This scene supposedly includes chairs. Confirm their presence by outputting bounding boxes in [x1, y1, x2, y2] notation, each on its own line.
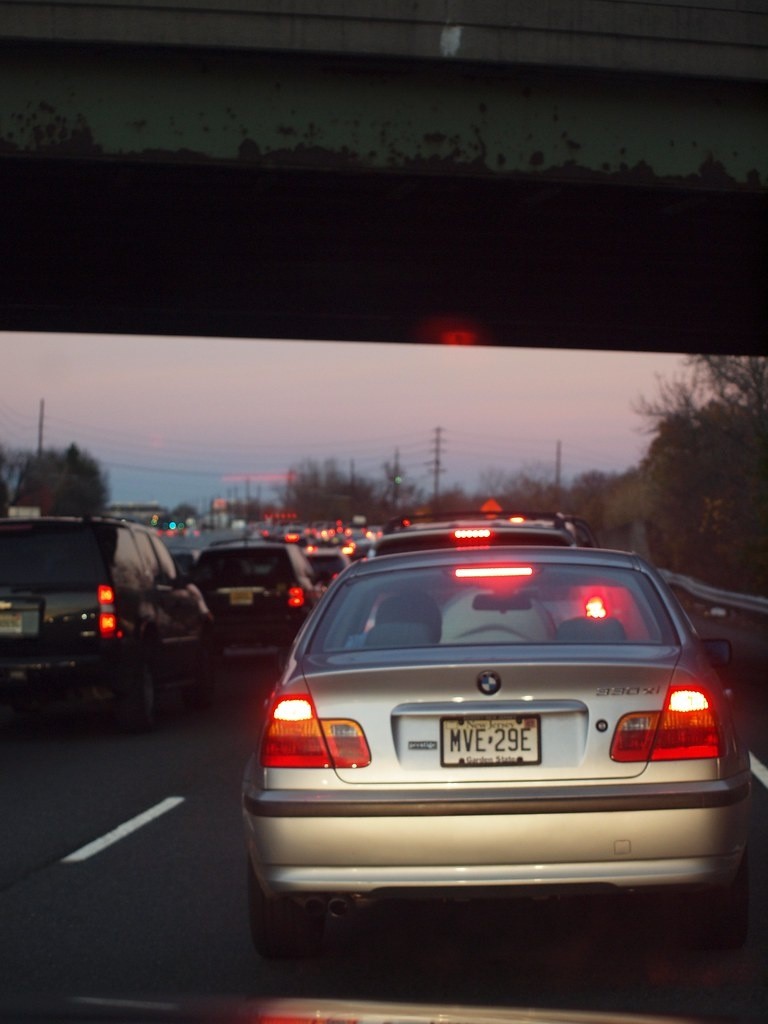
[555, 615, 627, 642]
[376, 592, 443, 646]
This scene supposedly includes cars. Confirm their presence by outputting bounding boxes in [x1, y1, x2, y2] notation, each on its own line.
[233, 543, 755, 947]
[112, 504, 589, 580]
[193, 542, 325, 666]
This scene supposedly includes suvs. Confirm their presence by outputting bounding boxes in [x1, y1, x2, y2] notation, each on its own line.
[2, 516, 218, 736]
